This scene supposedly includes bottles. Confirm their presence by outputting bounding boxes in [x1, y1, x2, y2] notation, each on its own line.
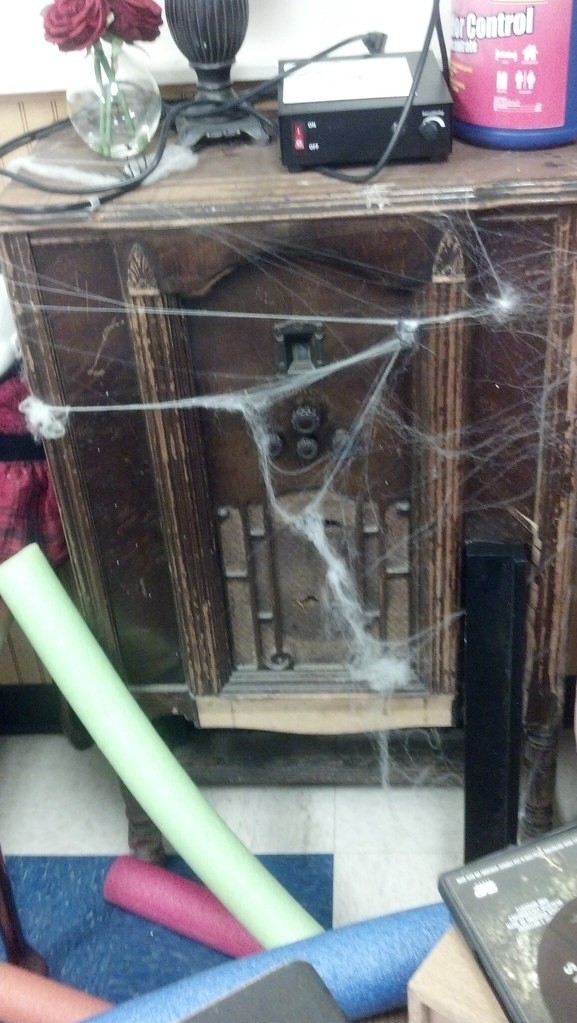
[442, 0, 577, 149]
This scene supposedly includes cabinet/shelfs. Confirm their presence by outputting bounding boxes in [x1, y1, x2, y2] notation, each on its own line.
[0, 139, 576, 868]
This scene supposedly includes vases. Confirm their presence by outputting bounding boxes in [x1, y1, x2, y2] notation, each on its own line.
[61, 57, 160, 157]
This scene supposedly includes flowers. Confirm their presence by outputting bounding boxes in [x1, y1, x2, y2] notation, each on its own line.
[43, 0, 162, 55]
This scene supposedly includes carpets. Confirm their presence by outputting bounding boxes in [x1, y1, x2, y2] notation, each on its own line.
[0, 855, 333, 1023]
